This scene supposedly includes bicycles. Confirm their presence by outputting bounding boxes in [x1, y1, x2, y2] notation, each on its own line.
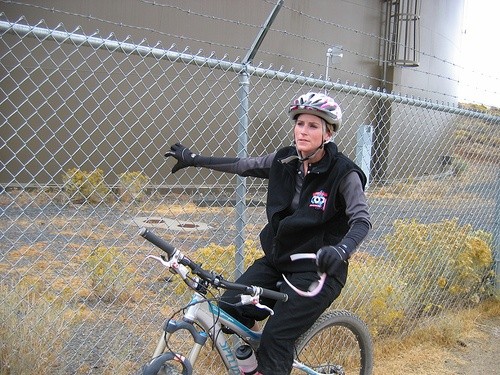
[134, 226, 374, 375]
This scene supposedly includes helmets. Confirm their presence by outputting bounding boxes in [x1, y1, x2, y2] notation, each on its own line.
[289, 92, 342, 132]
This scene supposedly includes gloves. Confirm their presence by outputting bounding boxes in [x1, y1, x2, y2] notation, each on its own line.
[316, 245, 347, 275]
[164, 143, 200, 174]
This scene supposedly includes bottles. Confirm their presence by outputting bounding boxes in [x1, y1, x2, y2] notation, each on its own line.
[235, 345, 258, 375]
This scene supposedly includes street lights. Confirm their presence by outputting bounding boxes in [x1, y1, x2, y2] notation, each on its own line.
[323, 44, 344, 95]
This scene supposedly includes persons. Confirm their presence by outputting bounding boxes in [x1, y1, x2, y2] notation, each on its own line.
[164, 92, 373, 375]
